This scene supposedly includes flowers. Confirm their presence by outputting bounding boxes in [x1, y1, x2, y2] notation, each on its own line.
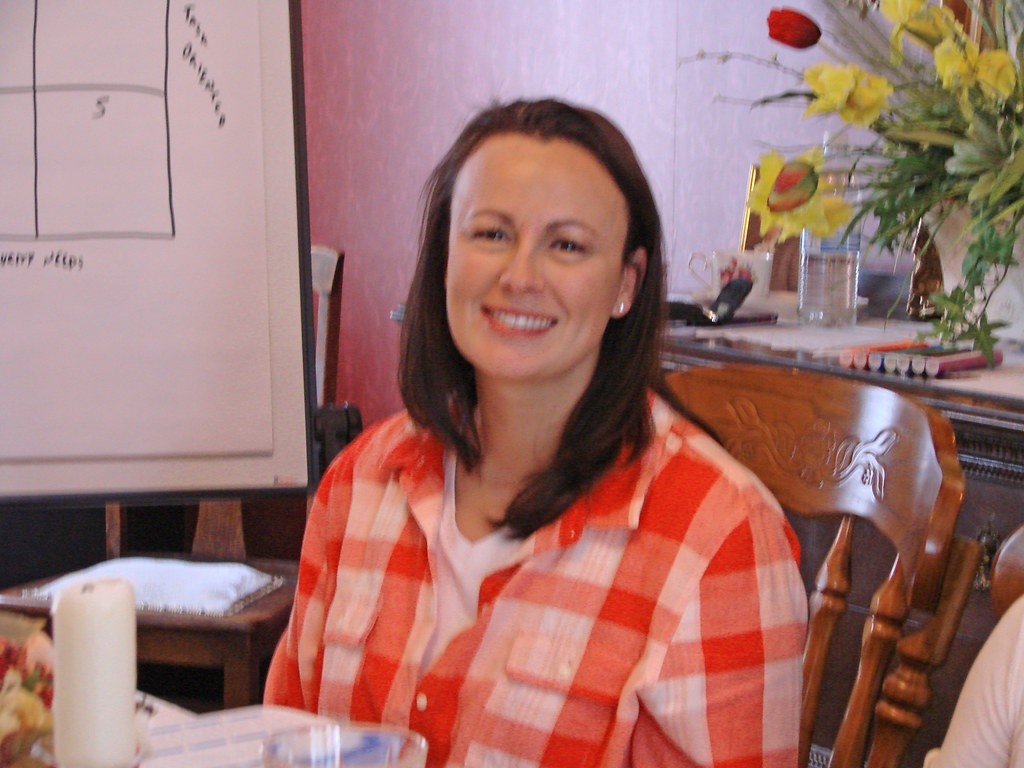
[749, 0, 1024, 373]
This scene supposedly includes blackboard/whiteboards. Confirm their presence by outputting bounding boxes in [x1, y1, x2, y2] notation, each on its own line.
[1, 0, 321, 504]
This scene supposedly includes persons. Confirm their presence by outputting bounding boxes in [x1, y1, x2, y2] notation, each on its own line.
[264, 97, 807, 768]
[930, 597, 1024, 768]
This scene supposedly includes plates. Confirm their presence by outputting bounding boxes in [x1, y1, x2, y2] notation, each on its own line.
[742, 290, 869, 319]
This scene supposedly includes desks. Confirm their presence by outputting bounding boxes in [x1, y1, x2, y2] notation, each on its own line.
[0, 560, 300, 711]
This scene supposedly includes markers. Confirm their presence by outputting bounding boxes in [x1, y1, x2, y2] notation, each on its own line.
[837, 339, 1002, 377]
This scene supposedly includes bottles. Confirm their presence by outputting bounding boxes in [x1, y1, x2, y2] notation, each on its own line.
[797, 130, 863, 327]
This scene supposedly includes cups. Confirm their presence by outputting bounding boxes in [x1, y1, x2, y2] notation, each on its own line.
[687, 249, 773, 298]
[260, 721, 429, 768]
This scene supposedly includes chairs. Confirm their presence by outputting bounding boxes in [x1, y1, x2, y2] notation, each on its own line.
[663, 366, 985, 768]
